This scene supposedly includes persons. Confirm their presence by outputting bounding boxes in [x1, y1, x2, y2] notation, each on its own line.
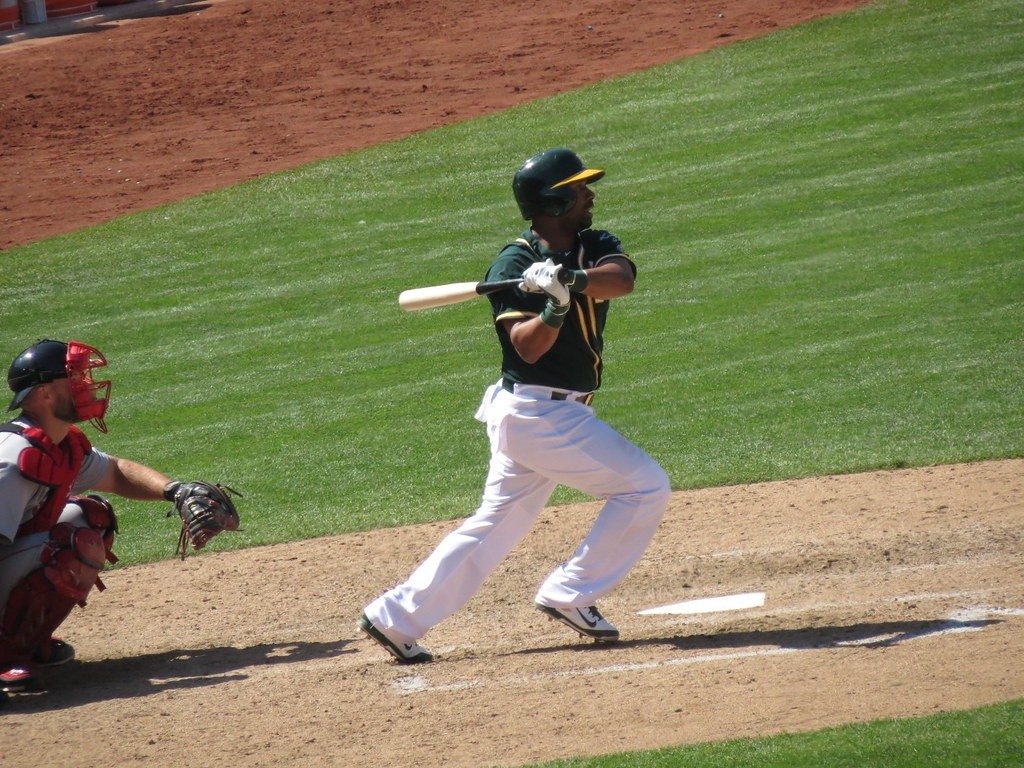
[354, 148, 671, 664]
[0, 338, 239, 700]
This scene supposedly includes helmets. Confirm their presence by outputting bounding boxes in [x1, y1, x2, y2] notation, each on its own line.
[7, 339, 83, 414]
[513, 147, 608, 220]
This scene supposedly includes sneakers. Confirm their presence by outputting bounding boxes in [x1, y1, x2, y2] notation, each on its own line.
[356, 610, 434, 663]
[535, 600, 620, 644]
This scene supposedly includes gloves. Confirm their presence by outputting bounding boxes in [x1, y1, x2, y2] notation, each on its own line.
[518, 260, 576, 296]
[534, 264, 571, 316]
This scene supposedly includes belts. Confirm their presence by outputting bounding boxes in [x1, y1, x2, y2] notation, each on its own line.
[502, 376, 594, 407]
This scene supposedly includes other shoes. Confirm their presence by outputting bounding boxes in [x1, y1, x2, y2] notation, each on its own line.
[21, 638, 75, 667]
[0, 663, 32, 692]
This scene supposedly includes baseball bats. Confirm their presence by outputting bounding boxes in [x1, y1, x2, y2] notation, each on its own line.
[397, 266, 573, 313]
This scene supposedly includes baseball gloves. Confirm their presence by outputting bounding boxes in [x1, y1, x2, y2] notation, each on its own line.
[166, 477, 241, 552]
